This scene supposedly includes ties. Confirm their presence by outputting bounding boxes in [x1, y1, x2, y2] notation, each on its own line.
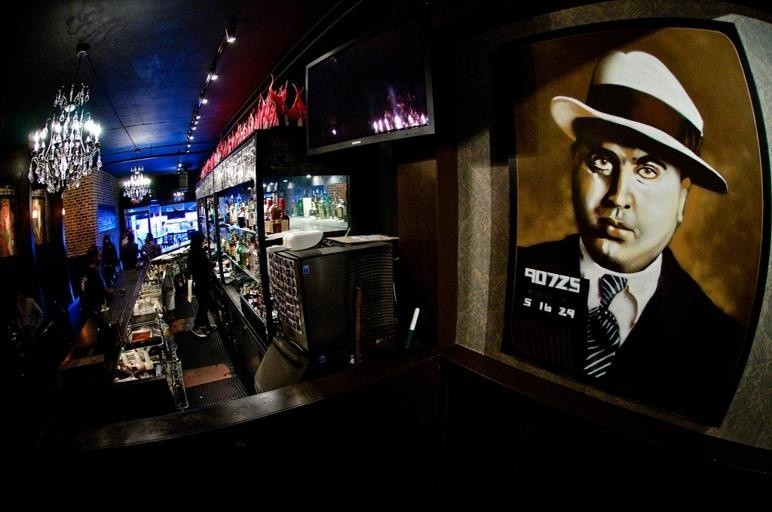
[584, 275, 628, 379]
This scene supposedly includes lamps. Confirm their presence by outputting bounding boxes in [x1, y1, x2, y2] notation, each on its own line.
[27, 42, 105, 196]
[176, 27, 237, 173]
[119, 148, 155, 206]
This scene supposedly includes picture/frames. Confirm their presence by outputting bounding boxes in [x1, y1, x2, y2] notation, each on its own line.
[497, 17, 772, 432]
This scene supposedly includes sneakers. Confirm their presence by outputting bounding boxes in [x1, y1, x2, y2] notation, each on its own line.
[192, 324, 216, 340]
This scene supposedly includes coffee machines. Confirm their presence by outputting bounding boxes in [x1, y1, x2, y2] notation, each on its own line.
[253, 221, 426, 398]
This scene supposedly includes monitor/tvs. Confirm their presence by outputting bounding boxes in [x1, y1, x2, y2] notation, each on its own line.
[303, 28, 439, 158]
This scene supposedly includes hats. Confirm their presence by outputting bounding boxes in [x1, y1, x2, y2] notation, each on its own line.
[550, 52, 730, 194]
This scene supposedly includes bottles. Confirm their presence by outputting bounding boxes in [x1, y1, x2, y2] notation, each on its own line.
[198, 176, 350, 325]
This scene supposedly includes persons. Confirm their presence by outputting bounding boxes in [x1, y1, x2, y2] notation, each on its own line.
[513, 47, 742, 426]
[81, 247, 112, 313]
[102, 234, 120, 284]
[16, 288, 46, 347]
[121, 227, 158, 267]
[183, 231, 220, 338]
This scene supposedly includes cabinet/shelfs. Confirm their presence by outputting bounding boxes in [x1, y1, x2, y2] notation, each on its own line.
[194, 124, 400, 397]
